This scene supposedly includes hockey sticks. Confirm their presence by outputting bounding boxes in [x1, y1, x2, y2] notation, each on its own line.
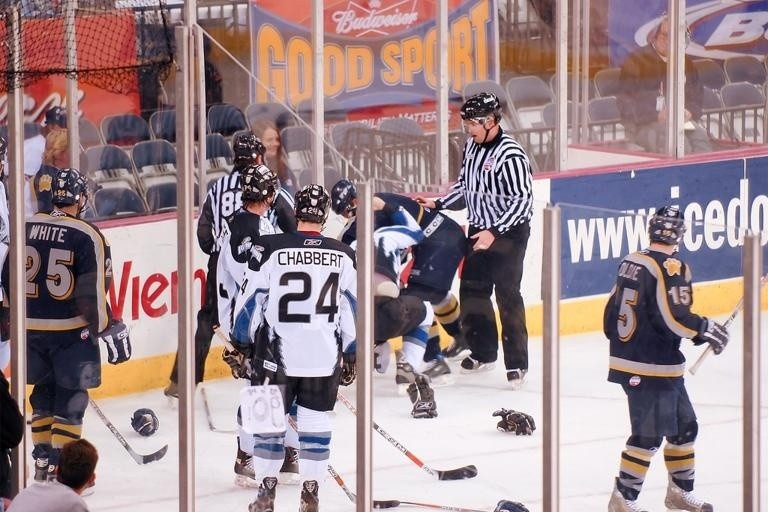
[88, 395, 168, 465]
[212, 324, 400, 508]
[201, 383, 240, 434]
[338, 393, 478, 482]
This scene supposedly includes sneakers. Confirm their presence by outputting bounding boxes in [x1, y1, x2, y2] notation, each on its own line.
[462, 354, 485, 370]
[233, 436, 258, 487]
[395, 338, 465, 418]
[279, 446, 301, 484]
[507, 369, 526, 380]
[248, 477, 278, 511]
[299, 480, 319, 511]
[165, 380, 179, 406]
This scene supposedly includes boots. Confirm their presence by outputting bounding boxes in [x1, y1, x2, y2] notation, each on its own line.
[608, 477, 647, 511]
[34, 457, 49, 480]
[665, 472, 712, 512]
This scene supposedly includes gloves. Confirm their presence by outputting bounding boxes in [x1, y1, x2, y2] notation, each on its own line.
[99, 319, 131, 364]
[691, 316, 728, 354]
[220, 344, 254, 380]
[493, 408, 536, 435]
[338, 361, 357, 386]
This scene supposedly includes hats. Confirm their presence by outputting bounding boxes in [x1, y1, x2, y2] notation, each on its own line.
[44, 107, 68, 129]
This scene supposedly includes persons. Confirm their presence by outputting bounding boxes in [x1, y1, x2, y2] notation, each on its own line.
[5, 439, 100, 512]
[615, 16, 712, 157]
[602, 205, 731, 512]
[223, 183, 360, 512]
[410, 91, 534, 381]
[161, 120, 467, 482]
[0, 370, 24, 512]
[1, 106, 131, 482]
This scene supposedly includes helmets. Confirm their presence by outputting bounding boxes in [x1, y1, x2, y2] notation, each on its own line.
[233, 93, 501, 224]
[648, 204, 687, 245]
[50, 168, 89, 204]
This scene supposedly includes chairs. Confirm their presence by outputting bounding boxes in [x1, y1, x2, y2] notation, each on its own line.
[0, 102, 246, 219]
[244, 95, 425, 200]
